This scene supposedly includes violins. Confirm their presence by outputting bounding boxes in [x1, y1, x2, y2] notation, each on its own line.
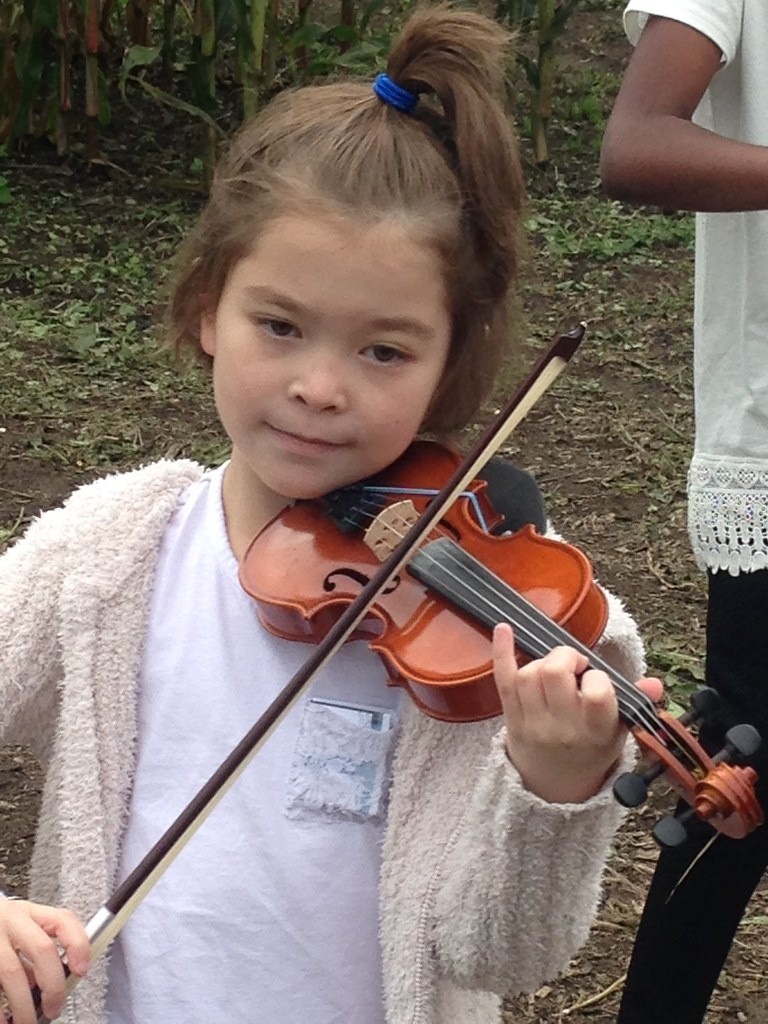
[235, 438, 767, 850]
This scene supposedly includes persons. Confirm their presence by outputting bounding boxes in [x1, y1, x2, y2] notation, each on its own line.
[0, 0, 666, 1023]
[588, 0, 768, 1024]
